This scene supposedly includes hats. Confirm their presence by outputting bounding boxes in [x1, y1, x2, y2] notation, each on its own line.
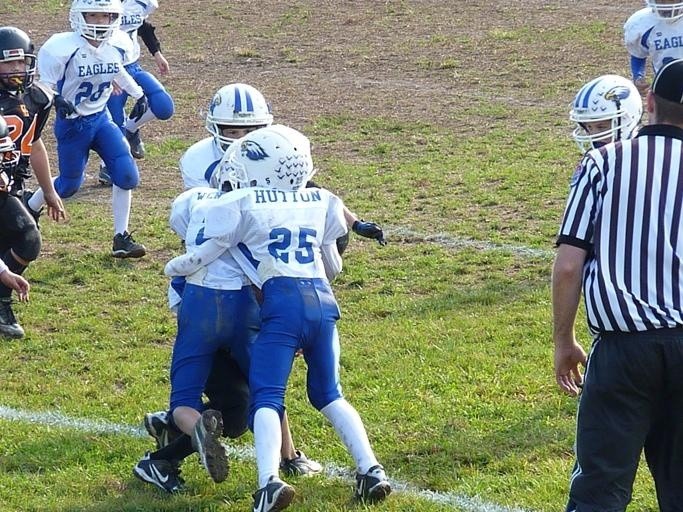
[652, 57, 683, 104]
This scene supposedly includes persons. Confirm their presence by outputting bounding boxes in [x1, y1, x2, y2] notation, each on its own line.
[0, 27, 66, 340]
[22, 0, 149, 257]
[98, 0, 174, 185]
[550, 61, 683, 512]
[0, 117, 30, 302]
[164, 124, 392, 512]
[169, 163, 322, 484]
[132, 82, 389, 493]
[623, 1, 682, 93]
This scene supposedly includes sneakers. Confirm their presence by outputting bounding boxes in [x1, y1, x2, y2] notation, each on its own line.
[112, 229, 147, 259]
[353, 462, 394, 507]
[250, 480, 297, 512]
[194, 407, 230, 485]
[124, 129, 146, 160]
[133, 456, 189, 499]
[281, 453, 327, 480]
[98, 161, 115, 187]
[144, 410, 174, 452]
[1, 302, 26, 340]
[19, 189, 40, 234]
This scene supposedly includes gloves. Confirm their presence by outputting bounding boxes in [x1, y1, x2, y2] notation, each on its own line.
[356, 221, 384, 246]
[129, 95, 149, 123]
[53, 94, 78, 120]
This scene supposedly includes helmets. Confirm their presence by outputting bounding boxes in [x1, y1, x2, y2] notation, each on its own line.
[0, 27, 37, 92]
[204, 84, 275, 152]
[70, 0, 125, 43]
[0, 116, 21, 189]
[646, 0, 683, 22]
[235, 124, 314, 189]
